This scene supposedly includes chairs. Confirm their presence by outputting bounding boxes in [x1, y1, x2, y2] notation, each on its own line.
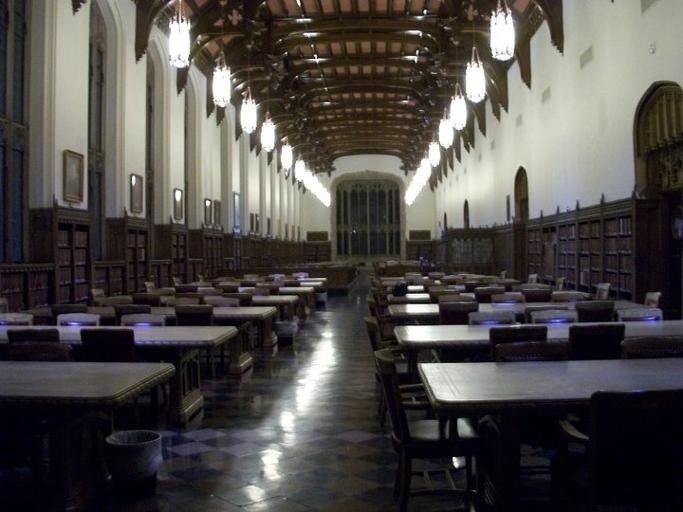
[165, 0, 308, 189]
[429, 0, 518, 176]
[1, 269, 328, 512]
[364, 255, 683, 511]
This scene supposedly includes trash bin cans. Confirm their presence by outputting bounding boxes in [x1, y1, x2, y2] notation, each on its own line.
[105, 429, 162, 500]
[313, 292, 329, 308]
[275, 321, 298, 346]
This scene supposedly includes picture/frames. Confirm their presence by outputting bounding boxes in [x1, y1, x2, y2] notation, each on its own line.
[62, 152, 302, 243]
